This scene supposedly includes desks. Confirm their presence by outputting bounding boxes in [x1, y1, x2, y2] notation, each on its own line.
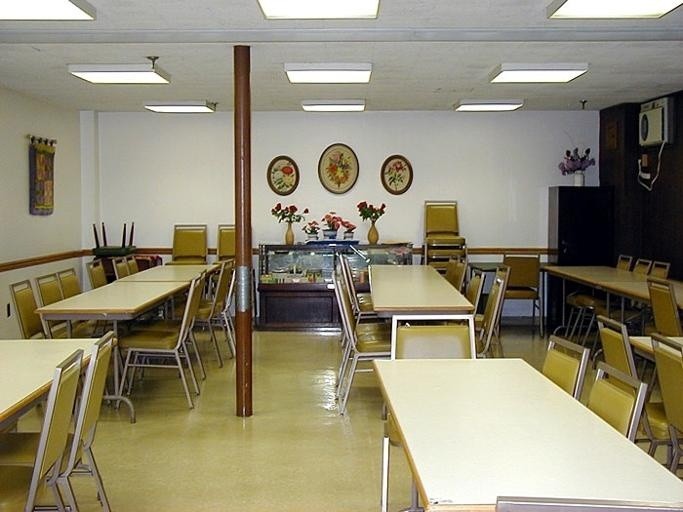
[1, 339, 118, 430]
[373, 358, 682, 511]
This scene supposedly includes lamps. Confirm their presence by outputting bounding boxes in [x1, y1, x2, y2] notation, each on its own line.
[66, 62, 171, 86]
[0, 1, 98, 23]
[299, 99, 366, 113]
[141, 99, 215, 114]
[281, 61, 373, 86]
[545, 0, 683, 21]
[254, 0, 381, 22]
[452, 98, 524, 112]
[487, 62, 589, 86]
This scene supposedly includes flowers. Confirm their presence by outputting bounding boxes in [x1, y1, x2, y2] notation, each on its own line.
[320, 210, 357, 233]
[557, 145, 595, 176]
[271, 165, 294, 192]
[384, 161, 408, 191]
[269, 202, 308, 224]
[324, 152, 352, 188]
[300, 221, 319, 236]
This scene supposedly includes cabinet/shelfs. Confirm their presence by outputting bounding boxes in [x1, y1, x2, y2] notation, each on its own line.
[255, 242, 414, 331]
[545, 183, 614, 337]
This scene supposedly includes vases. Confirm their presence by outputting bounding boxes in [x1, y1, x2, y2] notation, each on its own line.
[573, 170, 584, 187]
[322, 229, 337, 240]
[304, 233, 318, 242]
[284, 222, 295, 246]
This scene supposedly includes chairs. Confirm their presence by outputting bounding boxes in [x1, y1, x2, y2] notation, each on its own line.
[331, 251, 683, 479]
[9, 223, 238, 424]
[1, 329, 114, 512]
[381, 315, 476, 510]
[1, 349, 84, 511]
[422, 201, 469, 272]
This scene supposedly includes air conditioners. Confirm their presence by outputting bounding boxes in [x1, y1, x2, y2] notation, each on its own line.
[638, 96, 679, 151]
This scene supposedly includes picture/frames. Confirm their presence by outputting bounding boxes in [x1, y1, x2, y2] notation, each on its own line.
[379, 154, 414, 196]
[266, 155, 300, 197]
[316, 143, 360, 196]
